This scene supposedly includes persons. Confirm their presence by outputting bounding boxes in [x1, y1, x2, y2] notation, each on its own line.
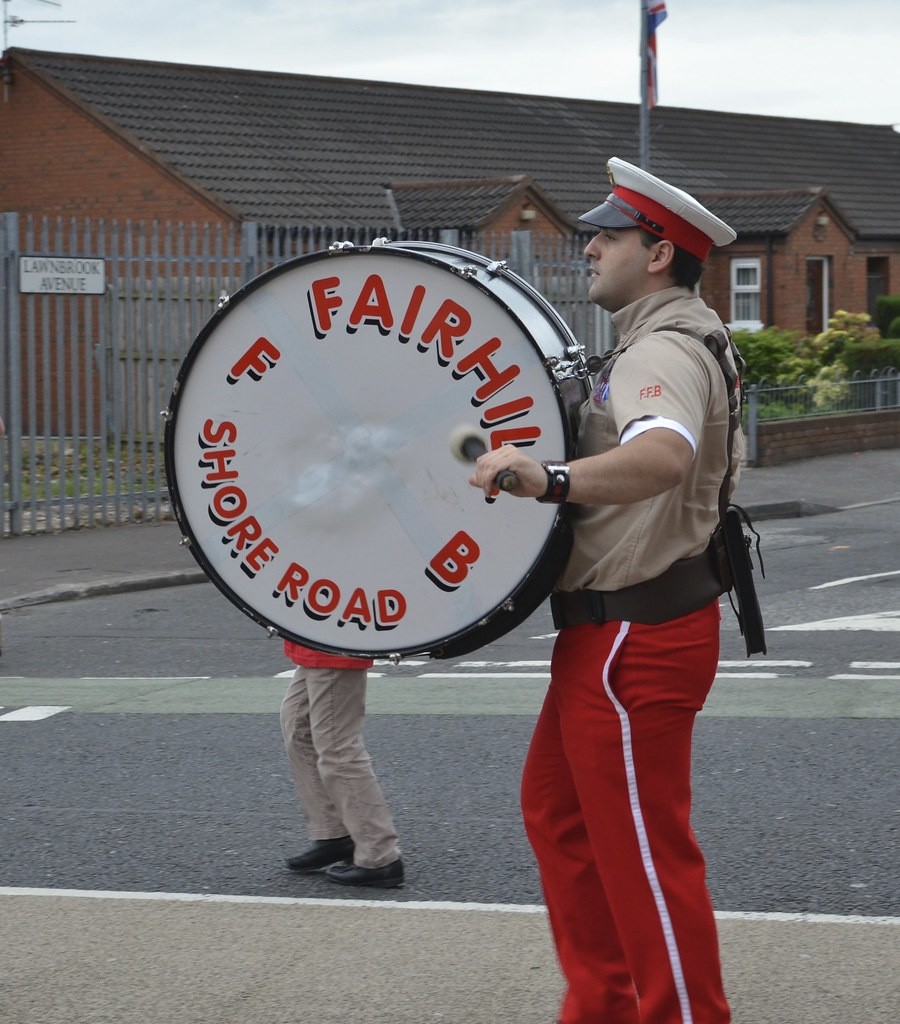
[469, 157, 743, 1024]
[281, 640, 404, 887]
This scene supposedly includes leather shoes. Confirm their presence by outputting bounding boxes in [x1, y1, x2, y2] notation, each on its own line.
[282, 837, 354, 873]
[324, 857, 404, 887]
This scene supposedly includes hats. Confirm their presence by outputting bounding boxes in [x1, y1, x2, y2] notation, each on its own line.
[578, 155, 737, 261]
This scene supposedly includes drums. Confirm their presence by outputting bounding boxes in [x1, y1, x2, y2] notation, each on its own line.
[161, 240, 596, 662]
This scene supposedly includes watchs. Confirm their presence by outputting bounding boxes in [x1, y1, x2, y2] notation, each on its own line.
[536, 460, 570, 504]
